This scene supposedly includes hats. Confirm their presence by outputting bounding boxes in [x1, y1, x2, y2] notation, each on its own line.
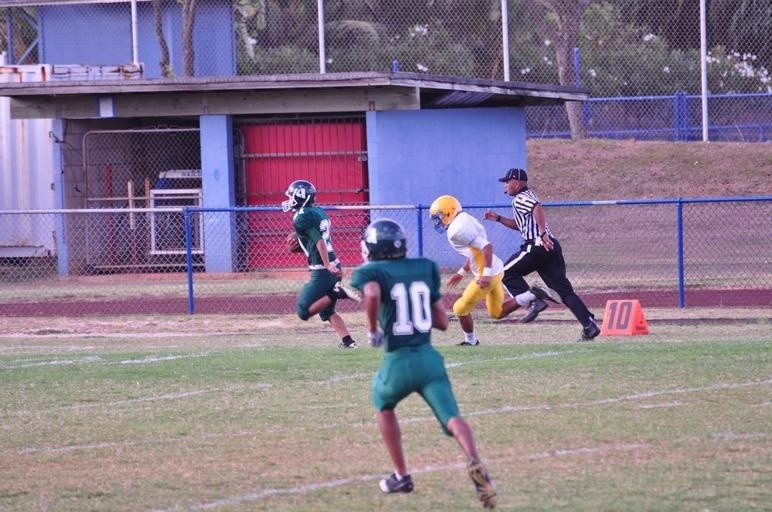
[498, 167, 528, 182]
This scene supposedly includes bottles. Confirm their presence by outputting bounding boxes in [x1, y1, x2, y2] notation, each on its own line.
[390, 57, 400, 71]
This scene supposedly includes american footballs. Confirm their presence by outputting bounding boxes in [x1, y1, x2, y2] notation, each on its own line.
[286, 232, 302, 252]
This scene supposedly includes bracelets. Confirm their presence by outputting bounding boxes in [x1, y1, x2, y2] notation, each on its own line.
[482, 266, 492, 277]
[497, 215, 501, 222]
[458, 269, 467, 277]
[540, 231, 546, 238]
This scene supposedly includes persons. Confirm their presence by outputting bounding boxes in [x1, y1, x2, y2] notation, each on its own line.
[350, 218, 497, 509]
[429, 194, 562, 347]
[484, 168, 600, 343]
[282, 179, 363, 348]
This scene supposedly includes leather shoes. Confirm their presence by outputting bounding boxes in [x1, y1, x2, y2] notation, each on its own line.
[577, 322, 601, 342]
[518, 299, 549, 323]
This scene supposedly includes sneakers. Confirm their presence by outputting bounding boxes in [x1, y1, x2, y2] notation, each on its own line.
[375, 473, 414, 495]
[461, 339, 480, 347]
[333, 280, 363, 303]
[465, 458, 499, 510]
[335, 340, 357, 350]
[531, 281, 562, 306]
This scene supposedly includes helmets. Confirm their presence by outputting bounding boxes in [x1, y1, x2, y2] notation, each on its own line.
[360, 217, 408, 260]
[428, 195, 463, 234]
[282, 179, 317, 213]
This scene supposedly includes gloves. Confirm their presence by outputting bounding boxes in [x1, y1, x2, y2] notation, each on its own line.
[366, 331, 383, 347]
